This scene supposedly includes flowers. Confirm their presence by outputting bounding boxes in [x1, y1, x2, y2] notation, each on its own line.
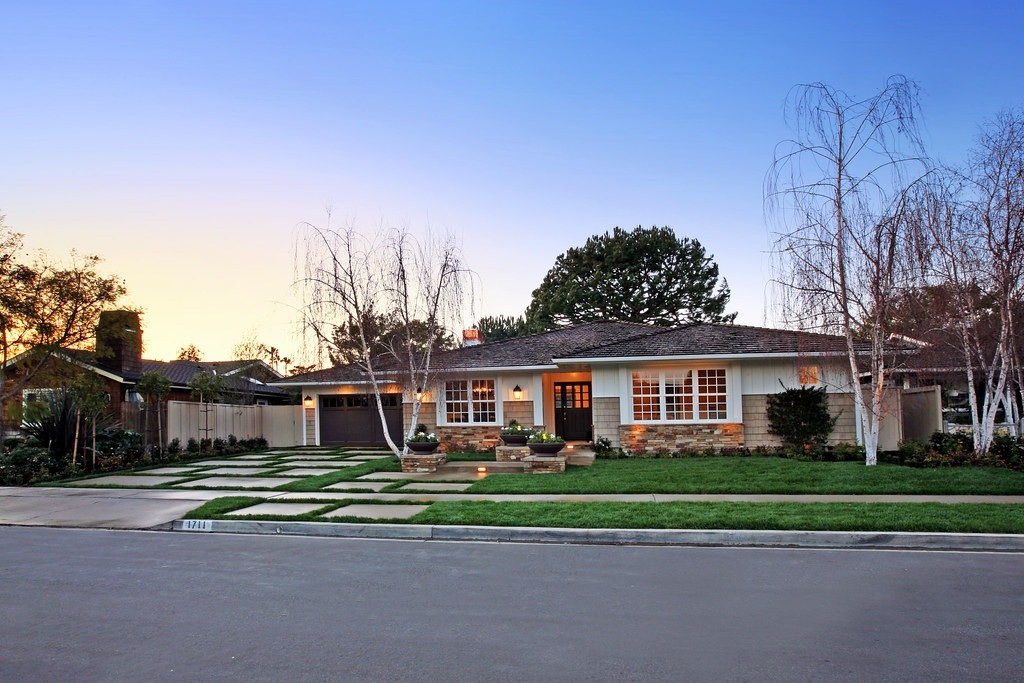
[406, 432, 437, 442]
[500, 425, 536, 436]
[528, 430, 565, 443]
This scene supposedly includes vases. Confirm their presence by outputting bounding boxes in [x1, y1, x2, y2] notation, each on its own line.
[406, 442, 440, 455]
[499, 435, 529, 446]
[526, 443, 567, 456]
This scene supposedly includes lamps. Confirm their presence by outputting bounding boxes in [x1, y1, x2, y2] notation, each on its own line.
[304, 394, 314, 407]
[414, 386, 424, 400]
[513, 384, 523, 399]
